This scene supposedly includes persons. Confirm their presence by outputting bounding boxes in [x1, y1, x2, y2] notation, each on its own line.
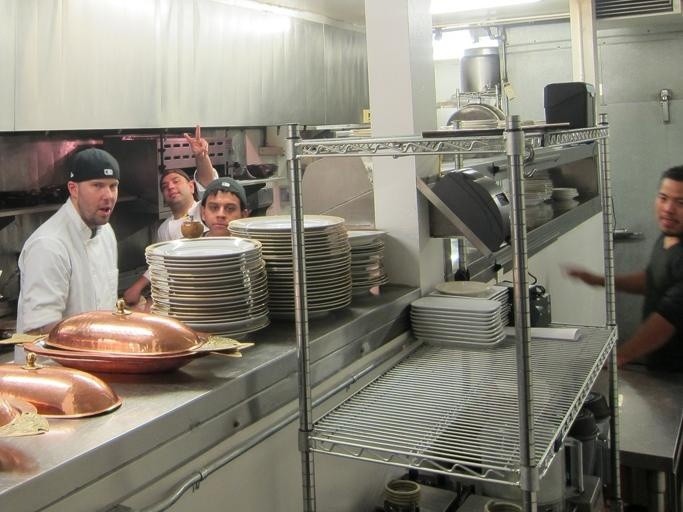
[122, 124, 219, 307]
[200, 177, 250, 237]
[564, 165, 683, 511]
[14, 148, 121, 366]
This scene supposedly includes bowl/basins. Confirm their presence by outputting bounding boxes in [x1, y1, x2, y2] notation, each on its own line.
[19, 337, 204, 375]
[44, 189, 67, 204]
[245, 163, 278, 179]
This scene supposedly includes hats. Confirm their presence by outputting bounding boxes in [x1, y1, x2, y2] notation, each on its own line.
[201, 176, 248, 207]
[66, 147, 120, 182]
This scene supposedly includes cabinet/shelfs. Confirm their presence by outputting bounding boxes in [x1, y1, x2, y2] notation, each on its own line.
[286, 111, 618, 512]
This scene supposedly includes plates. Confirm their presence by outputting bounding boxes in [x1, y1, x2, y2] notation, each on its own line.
[521, 199, 580, 228]
[549, 186, 579, 201]
[144, 234, 273, 343]
[227, 212, 353, 326]
[345, 228, 391, 297]
[407, 278, 510, 350]
[445, 103, 505, 127]
[500, 176, 553, 209]
